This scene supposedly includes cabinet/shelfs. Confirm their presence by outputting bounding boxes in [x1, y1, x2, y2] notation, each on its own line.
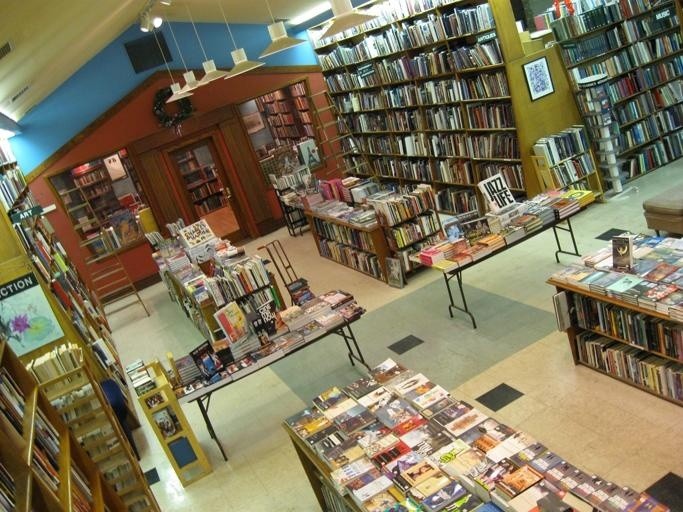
[304, 1, 605, 205]
[551, 3, 683, 193]
[280, 357, 672, 510]
[124, 357, 215, 488]
[270, 175, 446, 291]
[548, 230, 682, 405]
[0, 131, 162, 512]
[153, 239, 287, 340]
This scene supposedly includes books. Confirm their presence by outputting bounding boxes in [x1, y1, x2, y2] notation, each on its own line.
[0, 150, 152, 512]
[409, 190, 595, 273]
[550, 231, 683, 408]
[532, 0, 683, 192]
[386, 2, 525, 215]
[259, 166, 444, 295]
[147, 217, 360, 401]
[283, 359, 672, 512]
[315, 34, 390, 192]
[255, 82, 315, 147]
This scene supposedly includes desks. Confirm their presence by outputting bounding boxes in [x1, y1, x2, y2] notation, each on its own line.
[178, 289, 371, 465]
[409, 200, 590, 329]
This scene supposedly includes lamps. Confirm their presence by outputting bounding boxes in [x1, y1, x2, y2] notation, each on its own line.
[205, 2, 270, 84]
[175, 2, 228, 89]
[254, 2, 306, 62]
[139, 2, 201, 103]
